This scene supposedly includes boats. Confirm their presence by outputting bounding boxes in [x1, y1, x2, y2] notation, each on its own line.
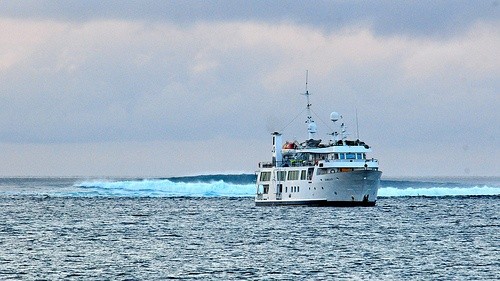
[253, 70, 382, 208]
